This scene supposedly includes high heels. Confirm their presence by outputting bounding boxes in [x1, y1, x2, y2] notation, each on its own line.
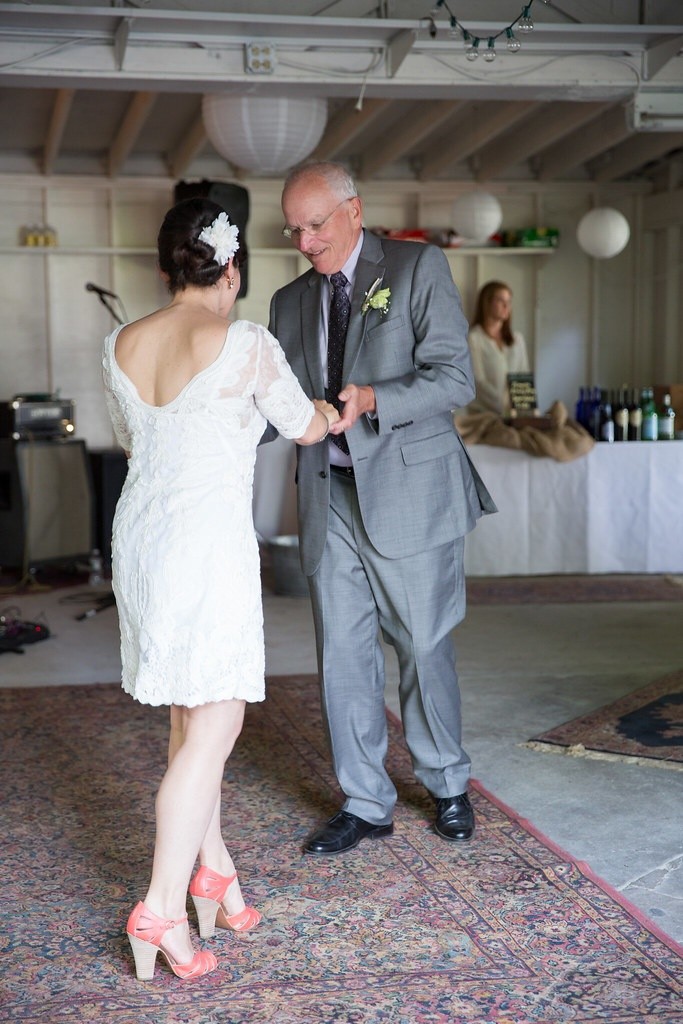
[126, 900, 219, 981]
[188, 866, 261, 939]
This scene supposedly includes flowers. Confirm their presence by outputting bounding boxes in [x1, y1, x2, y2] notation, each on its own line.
[358, 278, 391, 316]
[197, 209, 242, 265]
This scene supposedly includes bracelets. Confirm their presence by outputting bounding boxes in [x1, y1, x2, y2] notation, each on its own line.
[315, 407, 330, 443]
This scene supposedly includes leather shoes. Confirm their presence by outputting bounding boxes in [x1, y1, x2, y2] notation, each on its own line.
[302, 809, 393, 855]
[426, 788, 475, 842]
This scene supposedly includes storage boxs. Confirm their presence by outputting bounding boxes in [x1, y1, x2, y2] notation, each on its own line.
[513, 225, 560, 248]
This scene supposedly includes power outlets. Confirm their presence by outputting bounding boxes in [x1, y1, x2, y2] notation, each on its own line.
[245, 40, 276, 74]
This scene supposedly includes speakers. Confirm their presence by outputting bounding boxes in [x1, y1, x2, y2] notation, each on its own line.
[0, 437, 95, 574]
[172, 178, 251, 300]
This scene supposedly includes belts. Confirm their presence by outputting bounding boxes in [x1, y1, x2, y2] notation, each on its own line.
[328, 464, 355, 480]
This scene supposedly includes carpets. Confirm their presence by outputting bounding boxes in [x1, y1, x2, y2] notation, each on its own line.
[521, 666, 682, 770]
[0, 673, 683, 1024]
[461, 571, 683, 607]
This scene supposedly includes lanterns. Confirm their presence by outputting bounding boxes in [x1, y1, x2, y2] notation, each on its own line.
[577, 209, 628, 258]
[450, 190, 501, 239]
[202, 91, 327, 176]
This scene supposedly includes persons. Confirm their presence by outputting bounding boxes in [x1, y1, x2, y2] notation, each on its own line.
[257, 162, 500, 855]
[100, 198, 338, 982]
[469, 281, 528, 408]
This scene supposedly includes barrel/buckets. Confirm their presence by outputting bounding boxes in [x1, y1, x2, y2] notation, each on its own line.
[265, 533, 310, 596]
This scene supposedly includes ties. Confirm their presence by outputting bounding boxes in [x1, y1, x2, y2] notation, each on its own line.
[326, 272, 351, 456]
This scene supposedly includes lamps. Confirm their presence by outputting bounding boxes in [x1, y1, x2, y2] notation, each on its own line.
[203, 94, 329, 178]
[575, 204, 632, 260]
[451, 189, 504, 243]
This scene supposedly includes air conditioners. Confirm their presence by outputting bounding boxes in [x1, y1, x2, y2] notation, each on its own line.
[625, 92, 683, 131]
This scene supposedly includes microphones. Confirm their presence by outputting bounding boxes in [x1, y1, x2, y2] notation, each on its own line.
[86, 284, 116, 299]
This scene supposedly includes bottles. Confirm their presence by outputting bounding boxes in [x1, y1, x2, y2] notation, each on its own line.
[86, 548, 106, 586]
[575, 382, 675, 442]
[22, 222, 58, 247]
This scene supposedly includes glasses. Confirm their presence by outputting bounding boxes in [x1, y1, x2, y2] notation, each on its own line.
[281, 196, 357, 239]
